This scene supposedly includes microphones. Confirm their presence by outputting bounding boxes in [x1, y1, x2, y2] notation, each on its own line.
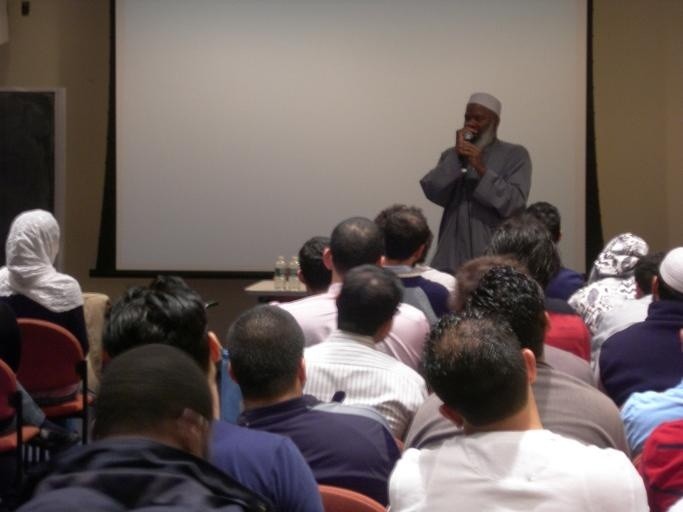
[460, 130, 474, 174]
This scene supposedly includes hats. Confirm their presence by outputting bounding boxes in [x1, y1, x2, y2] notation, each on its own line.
[658, 247, 682, 293]
[467, 92, 501, 117]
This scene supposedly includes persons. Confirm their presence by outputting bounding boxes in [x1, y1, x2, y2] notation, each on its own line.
[0, 205, 683, 512]
[418, 92, 531, 276]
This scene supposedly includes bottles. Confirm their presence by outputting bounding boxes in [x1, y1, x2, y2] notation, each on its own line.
[272, 256, 301, 294]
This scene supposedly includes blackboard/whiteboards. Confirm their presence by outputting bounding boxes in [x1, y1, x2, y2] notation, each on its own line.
[0, 85, 66, 276]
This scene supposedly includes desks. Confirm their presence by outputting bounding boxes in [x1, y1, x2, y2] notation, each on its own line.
[244, 281, 309, 302]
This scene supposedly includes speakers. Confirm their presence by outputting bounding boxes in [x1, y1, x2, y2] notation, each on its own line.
[0, 91, 54, 267]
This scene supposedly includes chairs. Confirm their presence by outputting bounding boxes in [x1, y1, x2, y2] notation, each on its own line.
[0, 317, 93, 498]
